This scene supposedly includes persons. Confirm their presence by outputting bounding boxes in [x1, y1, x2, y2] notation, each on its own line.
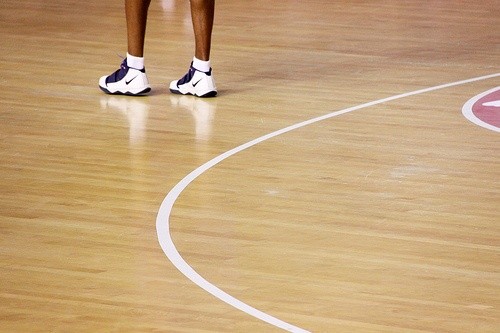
[98, 0, 218, 97]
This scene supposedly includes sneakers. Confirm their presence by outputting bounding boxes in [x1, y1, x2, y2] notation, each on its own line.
[169, 62, 218, 97]
[99, 58, 151, 95]
